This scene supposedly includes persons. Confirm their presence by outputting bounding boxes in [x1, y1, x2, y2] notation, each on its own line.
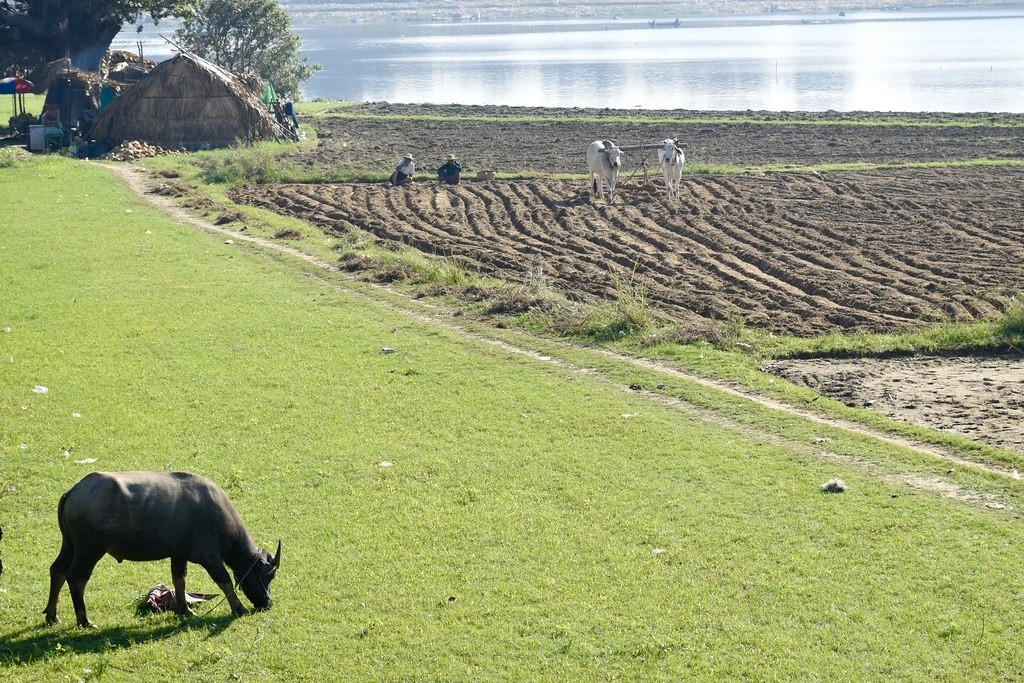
[390, 153, 415, 186]
[437, 154, 462, 185]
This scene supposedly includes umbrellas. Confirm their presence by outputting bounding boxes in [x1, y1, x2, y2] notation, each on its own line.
[0, 76, 38, 117]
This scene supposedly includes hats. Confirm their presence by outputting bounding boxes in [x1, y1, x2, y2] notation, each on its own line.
[403, 152, 414, 160]
[444, 154, 456, 160]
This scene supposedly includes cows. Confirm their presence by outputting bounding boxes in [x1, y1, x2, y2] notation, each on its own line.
[658, 138, 684, 199]
[43, 470, 281, 630]
[587, 140, 624, 204]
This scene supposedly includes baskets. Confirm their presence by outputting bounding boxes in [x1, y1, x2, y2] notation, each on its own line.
[477, 170, 496, 182]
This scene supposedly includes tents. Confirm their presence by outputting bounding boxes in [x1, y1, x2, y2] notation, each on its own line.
[89, 50, 280, 151]
